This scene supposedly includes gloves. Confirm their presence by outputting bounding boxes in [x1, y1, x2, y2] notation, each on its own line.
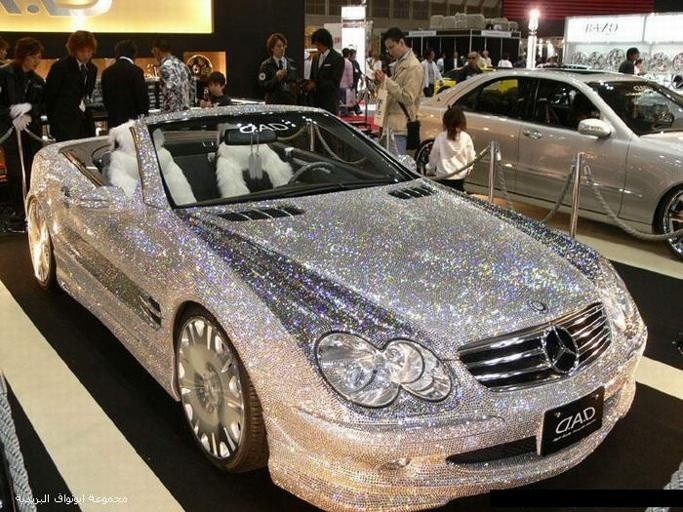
[13, 114, 31, 131]
[9, 102, 32, 118]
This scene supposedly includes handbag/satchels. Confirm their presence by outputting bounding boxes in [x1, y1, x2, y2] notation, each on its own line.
[405, 120, 420, 150]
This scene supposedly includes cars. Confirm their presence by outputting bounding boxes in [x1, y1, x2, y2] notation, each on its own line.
[404, 67, 683, 260]
[536, 62, 683, 129]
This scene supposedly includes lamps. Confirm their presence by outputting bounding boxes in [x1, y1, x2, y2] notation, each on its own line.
[525, 10, 539, 68]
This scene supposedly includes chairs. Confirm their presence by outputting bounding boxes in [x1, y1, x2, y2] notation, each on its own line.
[101, 119, 199, 206]
[536, 101, 570, 129]
[216, 121, 294, 199]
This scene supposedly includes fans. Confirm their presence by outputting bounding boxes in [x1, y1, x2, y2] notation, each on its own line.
[185, 55, 213, 104]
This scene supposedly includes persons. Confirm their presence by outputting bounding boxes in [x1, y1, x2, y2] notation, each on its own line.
[0, 29, 560, 226]
[634, 60, 642, 75]
[673, 75, 683, 89]
[618, 49, 638, 74]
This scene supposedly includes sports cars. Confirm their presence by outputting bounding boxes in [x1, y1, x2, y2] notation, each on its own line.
[20, 103, 648, 511]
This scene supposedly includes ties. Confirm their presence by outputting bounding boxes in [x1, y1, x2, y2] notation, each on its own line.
[80, 64, 87, 87]
[277, 59, 284, 89]
